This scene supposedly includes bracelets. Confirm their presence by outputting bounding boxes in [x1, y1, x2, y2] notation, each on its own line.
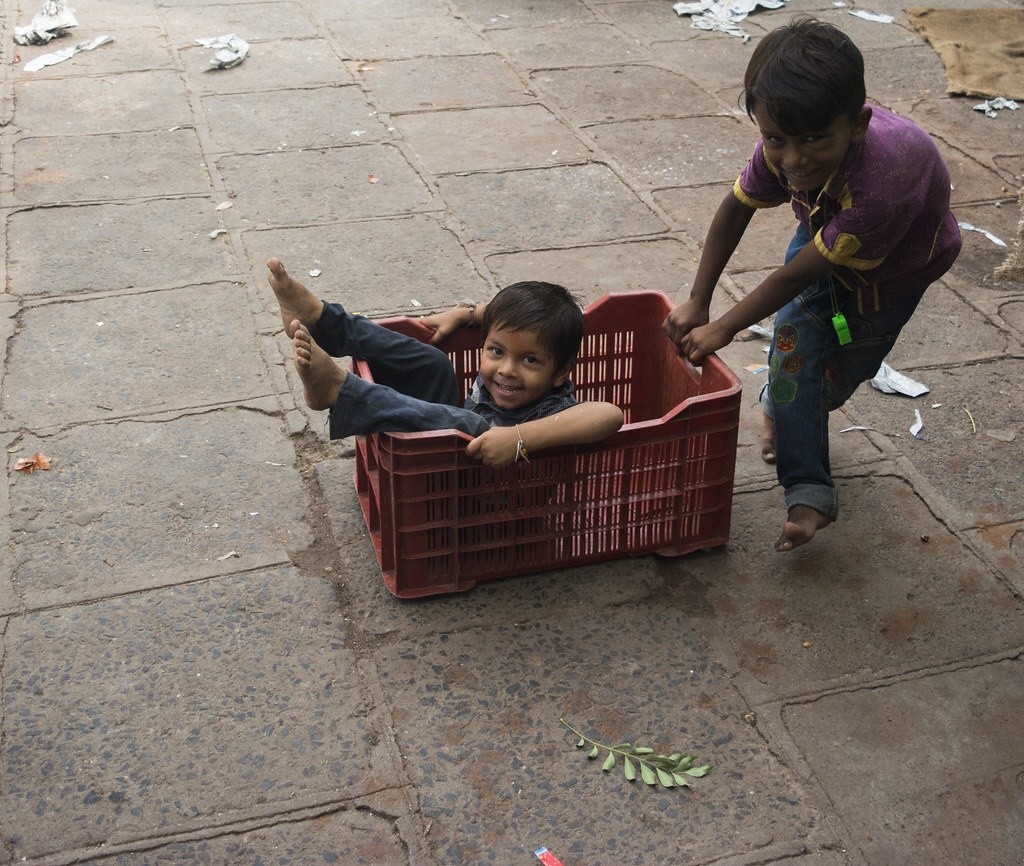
[458, 299, 476, 328]
[515, 424, 530, 464]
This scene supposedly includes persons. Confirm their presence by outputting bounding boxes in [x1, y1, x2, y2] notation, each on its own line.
[661, 12, 961, 553]
[265, 256, 624, 563]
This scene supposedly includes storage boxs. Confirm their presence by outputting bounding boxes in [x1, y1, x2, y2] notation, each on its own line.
[352, 289, 743, 601]
